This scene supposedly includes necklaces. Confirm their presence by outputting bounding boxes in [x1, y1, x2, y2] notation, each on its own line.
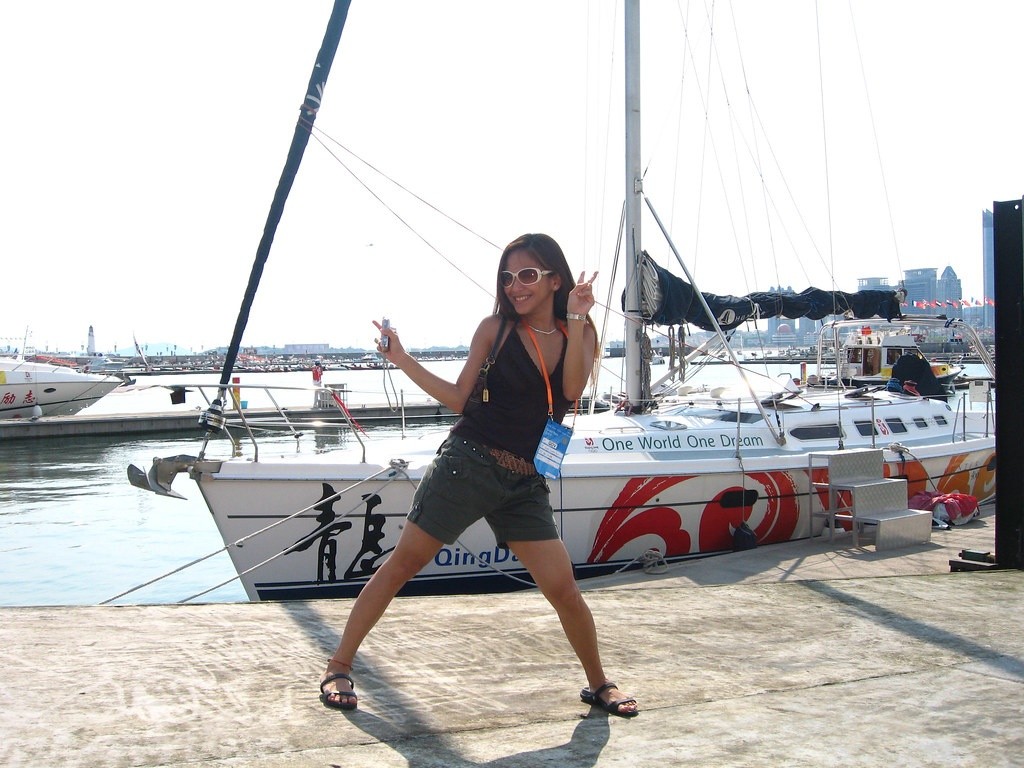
[527, 322, 559, 335]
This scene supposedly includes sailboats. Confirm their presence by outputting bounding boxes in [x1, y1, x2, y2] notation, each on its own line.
[146, 0, 995, 601]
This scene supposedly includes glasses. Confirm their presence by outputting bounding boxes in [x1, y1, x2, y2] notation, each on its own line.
[499, 267, 552, 289]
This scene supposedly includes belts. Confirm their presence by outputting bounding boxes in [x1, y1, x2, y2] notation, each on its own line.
[482, 443, 535, 476]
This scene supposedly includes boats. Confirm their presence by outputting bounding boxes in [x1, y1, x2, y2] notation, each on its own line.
[813, 326, 962, 393]
[80, 356, 126, 371]
[0, 356, 124, 419]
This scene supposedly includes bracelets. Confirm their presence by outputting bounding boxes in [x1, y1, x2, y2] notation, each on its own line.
[566, 313, 586, 321]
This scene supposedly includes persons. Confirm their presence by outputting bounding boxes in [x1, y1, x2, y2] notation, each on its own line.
[320, 234, 639, 717]
[312, 360, 322, 385]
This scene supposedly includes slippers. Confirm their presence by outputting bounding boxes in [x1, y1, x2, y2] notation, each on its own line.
[580, 682, 638, 717]
[320, 673, 358, 711]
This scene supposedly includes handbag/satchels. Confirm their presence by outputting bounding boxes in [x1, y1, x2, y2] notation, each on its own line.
[469, 316, 507, 405]
